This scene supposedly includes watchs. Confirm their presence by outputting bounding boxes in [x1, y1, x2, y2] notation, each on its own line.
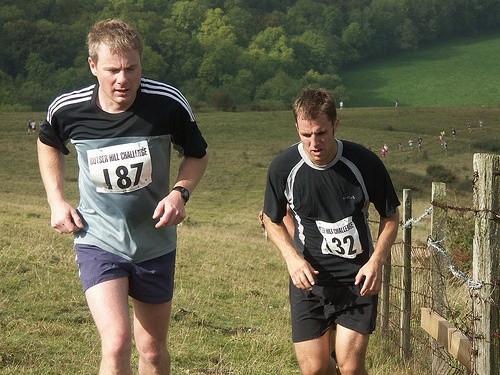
[173, 185, 190, 205]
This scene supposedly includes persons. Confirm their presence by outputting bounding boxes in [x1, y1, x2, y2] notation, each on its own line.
[27, 118, 37, 134]
[260, 86, 400, 375]
[380, 120, 484, 157]
[37, 18, 208, 375]
